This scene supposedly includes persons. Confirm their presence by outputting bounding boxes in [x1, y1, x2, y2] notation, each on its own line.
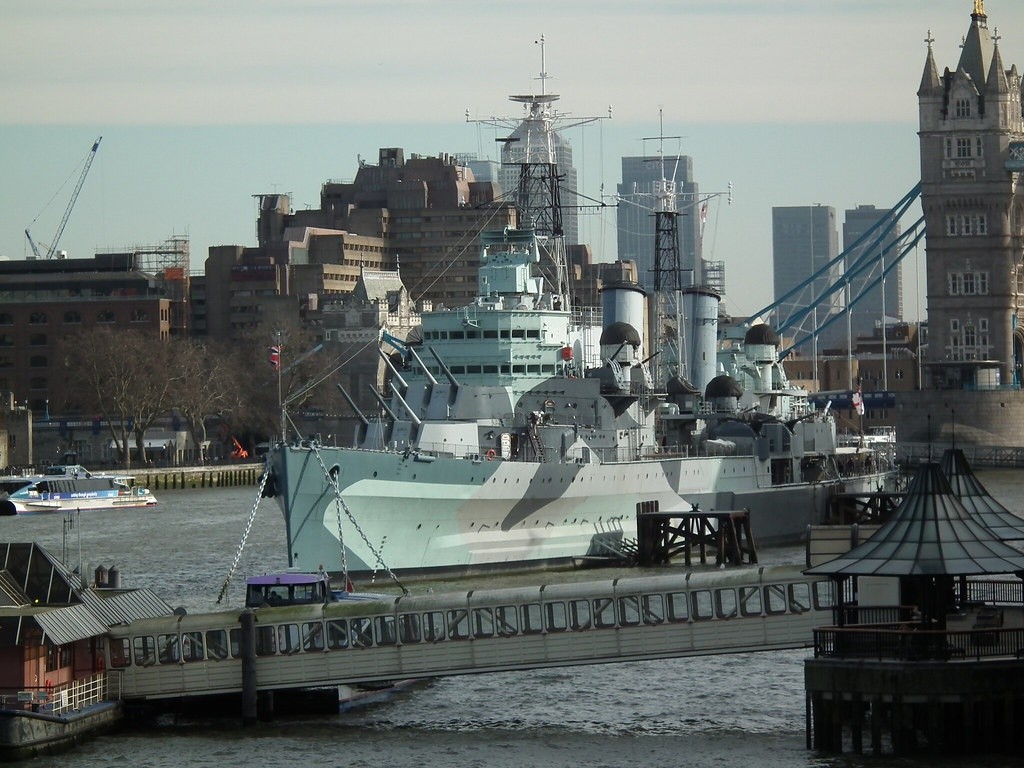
[269, 591, 283, 606]
[528, 413, 539, 436]
[318, 565, 329, 595]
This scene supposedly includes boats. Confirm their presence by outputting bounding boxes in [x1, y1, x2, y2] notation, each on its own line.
[0, 464, 158, 515]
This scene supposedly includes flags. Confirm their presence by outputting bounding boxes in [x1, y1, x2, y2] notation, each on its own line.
[853, 391, 864, 415]
[271, 345, 280, 369]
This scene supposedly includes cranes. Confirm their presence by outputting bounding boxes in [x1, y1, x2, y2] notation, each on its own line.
[24, 136, 104, 260]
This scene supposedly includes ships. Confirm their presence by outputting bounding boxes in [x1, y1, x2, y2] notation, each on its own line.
[258, 35, 904, 582]
